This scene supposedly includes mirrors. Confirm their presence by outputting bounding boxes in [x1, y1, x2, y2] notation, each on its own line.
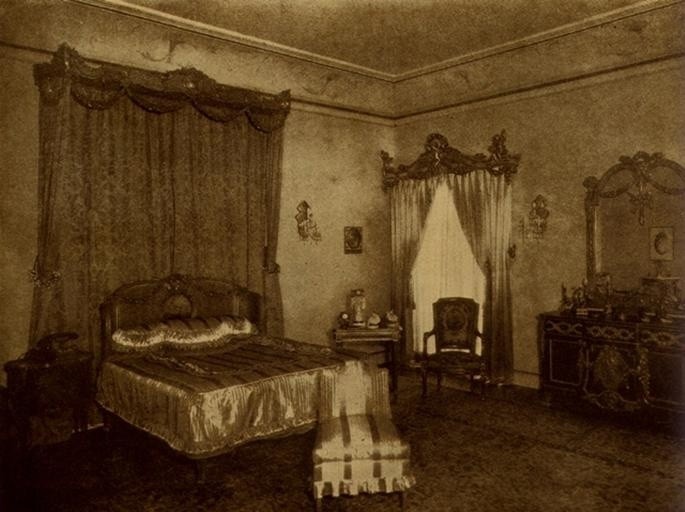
[583, 151, 685, 309]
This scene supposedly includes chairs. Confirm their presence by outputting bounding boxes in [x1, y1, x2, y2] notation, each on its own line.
[311, 368, 419, 511]
[424, 298, 489, 400]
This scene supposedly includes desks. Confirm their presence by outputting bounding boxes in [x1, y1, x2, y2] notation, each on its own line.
[332, 327, 403, 376]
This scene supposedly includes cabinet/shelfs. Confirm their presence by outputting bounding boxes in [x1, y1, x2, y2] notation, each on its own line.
[535, 309, 685, 438]
[5, 352, 94, 465]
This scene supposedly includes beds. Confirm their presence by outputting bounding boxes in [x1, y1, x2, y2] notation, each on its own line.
[96, 274, 369, 482]
